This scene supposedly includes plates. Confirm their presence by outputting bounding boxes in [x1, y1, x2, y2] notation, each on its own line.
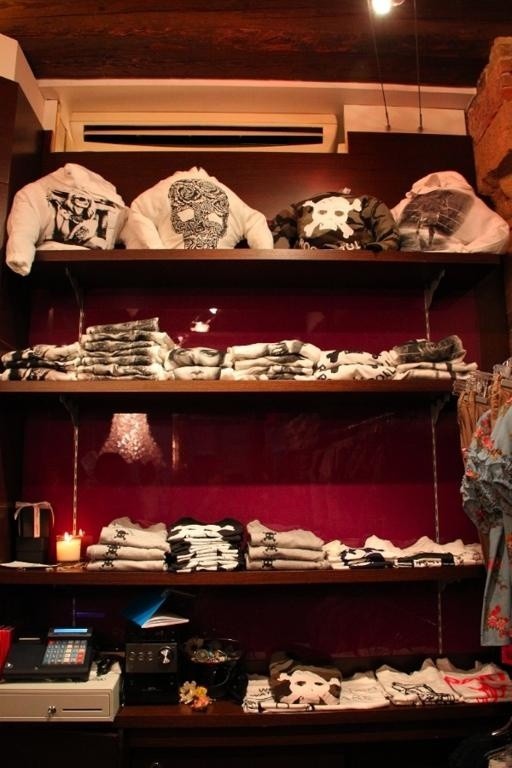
[0, 130, 512, 768]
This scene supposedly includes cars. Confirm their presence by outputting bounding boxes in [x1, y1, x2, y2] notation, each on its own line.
[55, 534, 83, 561]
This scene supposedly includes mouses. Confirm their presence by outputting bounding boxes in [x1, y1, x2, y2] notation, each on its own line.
[451, 357, 512, 405]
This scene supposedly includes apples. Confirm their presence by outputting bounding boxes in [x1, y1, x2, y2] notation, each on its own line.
[56, 532, 80, 561]
